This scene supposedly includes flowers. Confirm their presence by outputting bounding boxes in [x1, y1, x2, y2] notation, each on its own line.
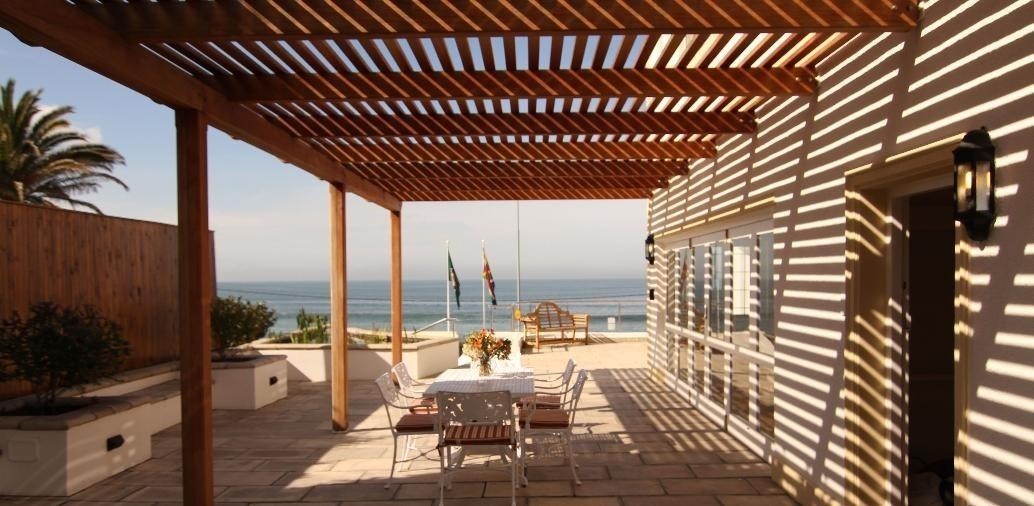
[462, 328, 511, 360]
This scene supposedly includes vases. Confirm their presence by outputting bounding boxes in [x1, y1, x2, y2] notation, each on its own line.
[478, 363, 492, 376]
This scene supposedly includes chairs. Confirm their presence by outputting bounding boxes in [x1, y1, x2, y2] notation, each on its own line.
[375, 372, 440, 489]
[519, 369, 587, 486]
[392, 362, 438, 413]
[438, 390, 517, 506]
[518, 358, 576, 406]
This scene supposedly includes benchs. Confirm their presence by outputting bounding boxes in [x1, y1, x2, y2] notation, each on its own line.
[522, 302, 589, 350]
[116, 374, 209, 446]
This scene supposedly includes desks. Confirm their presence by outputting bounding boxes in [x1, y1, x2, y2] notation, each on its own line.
[422, 368, 534, 398]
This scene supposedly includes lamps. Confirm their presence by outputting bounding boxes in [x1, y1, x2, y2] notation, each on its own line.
[645, 234, 655, 264]
[950, 127, 995, 239]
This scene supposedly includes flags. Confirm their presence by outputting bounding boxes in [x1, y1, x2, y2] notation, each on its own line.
[481, 254, 497, 305]
[448, 253, 460, 309]
